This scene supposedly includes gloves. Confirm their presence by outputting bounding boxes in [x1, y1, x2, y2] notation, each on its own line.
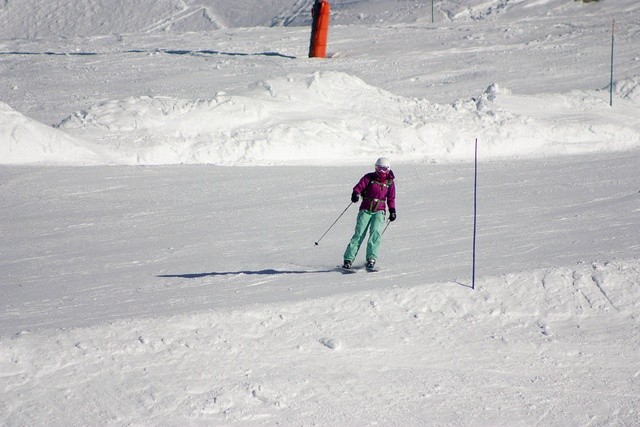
[389, 208, 396, 222]
[351, 192, 359, 203]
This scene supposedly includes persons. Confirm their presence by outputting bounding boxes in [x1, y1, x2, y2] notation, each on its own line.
[343, 158, 396, 269]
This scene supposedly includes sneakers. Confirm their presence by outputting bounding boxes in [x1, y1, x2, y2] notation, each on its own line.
[367, 259, 376, 269]
[342, 260, 351, 268]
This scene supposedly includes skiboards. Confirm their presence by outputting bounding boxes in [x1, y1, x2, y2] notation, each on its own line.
[336, 264, 379, 273]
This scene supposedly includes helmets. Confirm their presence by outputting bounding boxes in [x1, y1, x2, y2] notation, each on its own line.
[375, 157, 390, 178]
